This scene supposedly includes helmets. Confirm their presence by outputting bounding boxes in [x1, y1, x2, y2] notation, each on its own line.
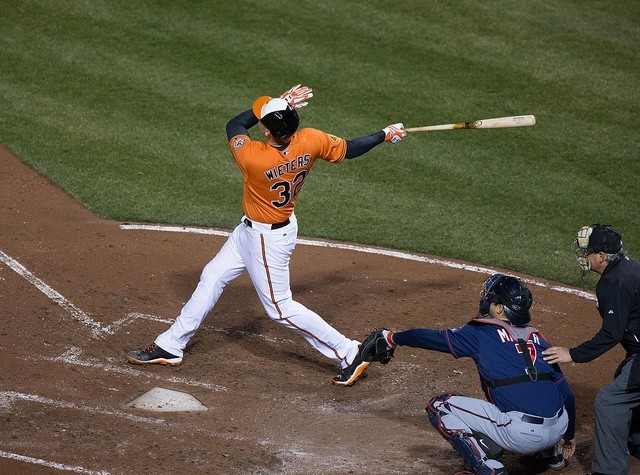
[252, 95, 299, 140]
[477, 274, 532, 326]
[574, 224, 623, 280]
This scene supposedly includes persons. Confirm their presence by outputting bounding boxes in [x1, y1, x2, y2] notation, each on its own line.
[359, 273, 576, 475]
[125, 83, 407, 386]
[542, 223, 640, 475]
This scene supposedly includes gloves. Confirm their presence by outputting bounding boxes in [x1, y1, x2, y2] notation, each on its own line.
[279, 83, 313, 109]
[382, 123, 407, 145]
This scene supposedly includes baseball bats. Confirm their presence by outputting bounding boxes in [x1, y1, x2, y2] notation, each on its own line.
[404, 114, 536, 132]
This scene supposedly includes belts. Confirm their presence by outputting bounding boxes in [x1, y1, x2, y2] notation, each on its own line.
[243, 212, 290, 230]
[521, 408, 564, 424]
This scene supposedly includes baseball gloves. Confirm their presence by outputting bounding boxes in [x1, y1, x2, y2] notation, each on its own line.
[359, 328, 395, 363]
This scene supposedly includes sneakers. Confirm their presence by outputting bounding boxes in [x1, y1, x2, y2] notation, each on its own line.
[127, 343, 182, 367]
[332, 352, 371, 386]
[535, 452, 565, 470]
